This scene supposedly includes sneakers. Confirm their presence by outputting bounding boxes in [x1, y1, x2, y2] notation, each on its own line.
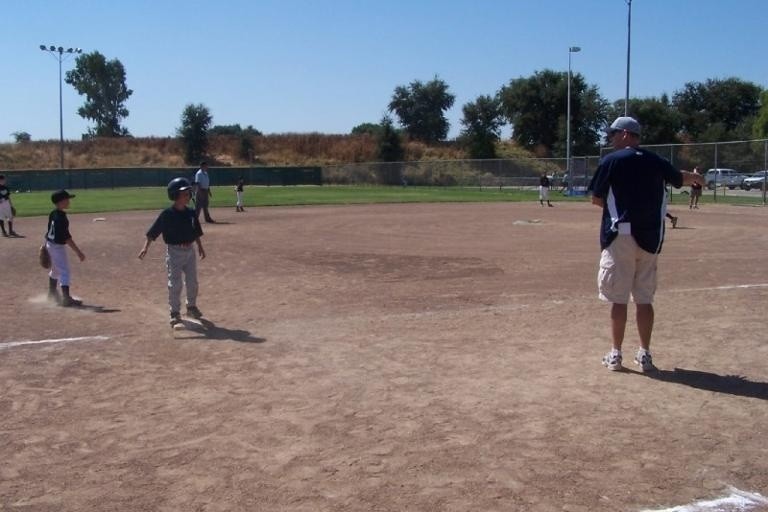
[540, 204, 554, 207]
[205, 206, 244, 223]
[186, 306, 202, 319]
[2, 232, 15, 237]
[169, 312, 181, 324]
[633, 355, 654, 370]
[601, 354, 623, 371]
[670, 206, 701, 229]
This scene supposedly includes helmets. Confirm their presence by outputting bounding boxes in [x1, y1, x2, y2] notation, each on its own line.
[167, 177, 192, 200]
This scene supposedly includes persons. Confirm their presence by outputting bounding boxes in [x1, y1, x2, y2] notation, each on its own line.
[588, 116, 704, 371]
[0, 172, 18, 236]
[139, 177, 206, 325]
[45, 189, 87, 307]
[663, 187, 678, 229]
[539, 170, 554, 208]
[193, 161, 215, 225]
[232, 176, 246, 212]
[689, 168, 702, 210]
[559, 170, 569, 194]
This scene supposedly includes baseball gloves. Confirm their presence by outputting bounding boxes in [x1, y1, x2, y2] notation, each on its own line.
[39, 246, 50, 269]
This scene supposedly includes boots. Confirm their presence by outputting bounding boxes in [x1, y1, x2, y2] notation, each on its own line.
[61, 285, 82, 306]
[47, 276, 59, 299]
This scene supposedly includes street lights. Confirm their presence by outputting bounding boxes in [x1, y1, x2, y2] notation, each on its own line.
[565, 46, 580, 176]
[36, 44, 85, 167]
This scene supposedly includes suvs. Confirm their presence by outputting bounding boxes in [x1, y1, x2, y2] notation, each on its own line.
[704, 167, 753, 192]
[744, 170, 767, 192]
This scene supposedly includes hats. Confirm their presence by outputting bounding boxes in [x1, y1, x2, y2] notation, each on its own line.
[51, 189, 76, 203]
[603, 117, 643, 136]
